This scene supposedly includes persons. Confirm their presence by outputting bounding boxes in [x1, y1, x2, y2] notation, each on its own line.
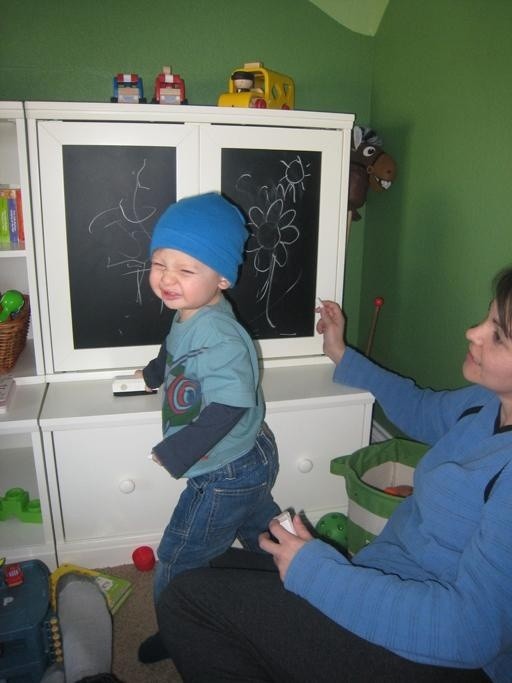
[133, 192, 282, 662]
[155, 269, 512, 683]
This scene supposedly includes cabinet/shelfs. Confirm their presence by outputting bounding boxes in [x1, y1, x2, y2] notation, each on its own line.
[37, 363, 379, 571]
[2, 99, 46, 381]
[23, 92, 361, 384]
[1, 380, 59, 572]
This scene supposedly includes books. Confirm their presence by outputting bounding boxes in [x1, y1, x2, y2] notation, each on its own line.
[47, 562, 133, 616]
[0, 378, 17, 413]
[0, 188, 24, 244]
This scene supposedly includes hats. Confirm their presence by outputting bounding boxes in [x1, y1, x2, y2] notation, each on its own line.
[147, 191, 250, 292]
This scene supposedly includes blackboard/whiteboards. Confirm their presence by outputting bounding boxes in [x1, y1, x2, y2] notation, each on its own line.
[199, 125, 343, 358]
[37, 120, 200, 373]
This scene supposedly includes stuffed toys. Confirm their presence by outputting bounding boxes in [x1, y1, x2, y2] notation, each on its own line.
[348, 125, 397, 221]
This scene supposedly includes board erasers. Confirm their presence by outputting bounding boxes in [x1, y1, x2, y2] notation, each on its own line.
[112, 376, 158, 396]
